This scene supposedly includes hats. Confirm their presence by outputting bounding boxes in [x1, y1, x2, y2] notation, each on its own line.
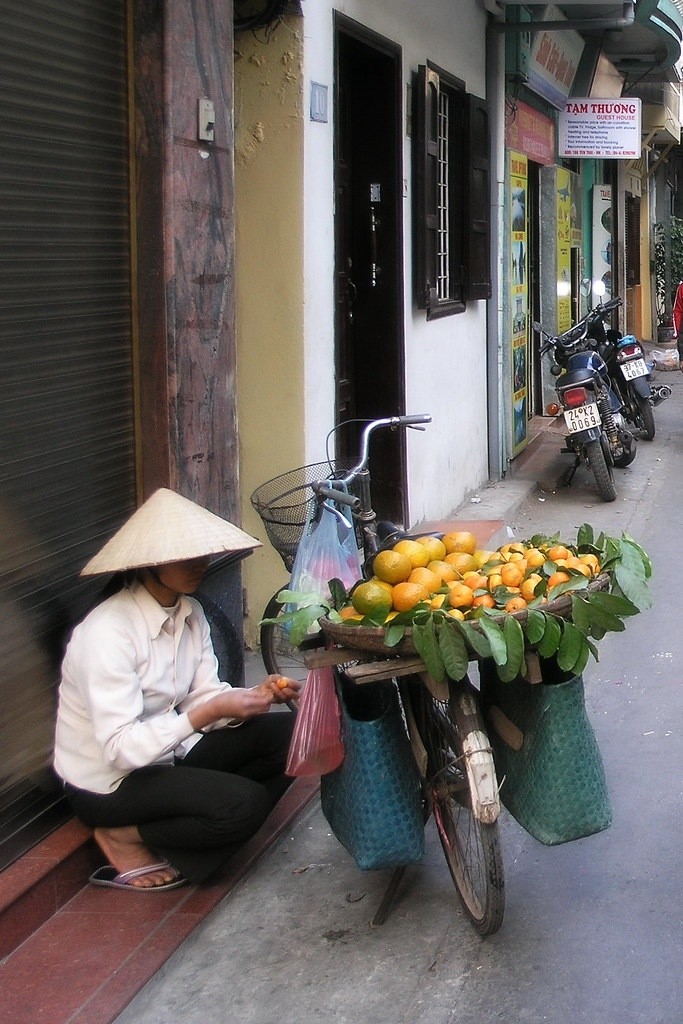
[80, 488, 265, 575]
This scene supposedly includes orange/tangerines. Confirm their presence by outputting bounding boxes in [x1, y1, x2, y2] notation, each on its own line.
[338, 531, 601, 620]
[276, 676, 289, 688]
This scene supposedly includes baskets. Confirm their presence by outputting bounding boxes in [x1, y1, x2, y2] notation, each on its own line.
[314, 572, 609, 655]
[249, 458, 372, 573]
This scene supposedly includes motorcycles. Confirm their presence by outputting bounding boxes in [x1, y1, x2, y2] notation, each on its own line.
[530, 277, 672, 502]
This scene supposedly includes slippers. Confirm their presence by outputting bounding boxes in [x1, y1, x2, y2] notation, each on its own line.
[90, 859, 187, 892]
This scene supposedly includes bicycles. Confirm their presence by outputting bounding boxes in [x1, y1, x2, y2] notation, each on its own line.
[249, 413, 506, 937]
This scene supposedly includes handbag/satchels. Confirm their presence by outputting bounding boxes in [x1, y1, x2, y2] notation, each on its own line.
[319, 669, 424, 872]
[285, 666, 345, 776]
[477, 652, 612, 847]
[650, 348, 680, 371]
[277, 478, 368, 647]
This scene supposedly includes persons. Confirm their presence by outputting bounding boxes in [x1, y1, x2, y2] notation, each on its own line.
[672, 281, 683, 372]
[55, 486, 302, 893]
